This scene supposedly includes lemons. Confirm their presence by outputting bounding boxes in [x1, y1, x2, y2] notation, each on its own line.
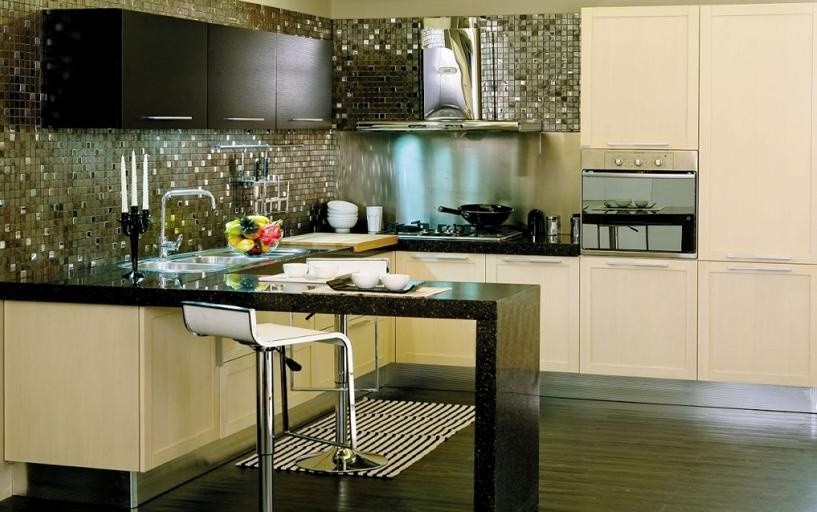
[225, 214, 270, 254]
[222, 273, 268, 292]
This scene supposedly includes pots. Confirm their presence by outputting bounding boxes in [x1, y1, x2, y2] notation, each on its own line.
[438, 203, 516, 226]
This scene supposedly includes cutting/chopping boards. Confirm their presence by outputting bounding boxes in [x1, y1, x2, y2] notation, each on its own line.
[281, 232, 399, 253]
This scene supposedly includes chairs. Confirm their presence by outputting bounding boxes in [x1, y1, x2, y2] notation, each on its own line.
[290, 253, 397, 473]
[179, 297, 353, 509]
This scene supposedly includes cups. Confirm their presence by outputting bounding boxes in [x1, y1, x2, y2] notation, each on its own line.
[229, 152, 246, 177]
[365, 205, 383, 232]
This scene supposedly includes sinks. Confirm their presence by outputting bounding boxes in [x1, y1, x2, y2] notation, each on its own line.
[117, 258, 230, 280]
[165, 252, 269, 272]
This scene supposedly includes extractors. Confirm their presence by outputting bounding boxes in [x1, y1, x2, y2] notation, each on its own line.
[353, 16, 543, 131]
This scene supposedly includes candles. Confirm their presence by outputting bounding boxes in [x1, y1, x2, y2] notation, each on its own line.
[120, 154, 128, 213]
[131, 150, 138, 206]
[142, 153, 149, 209]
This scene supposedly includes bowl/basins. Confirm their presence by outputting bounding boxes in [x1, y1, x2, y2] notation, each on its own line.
[327, 199, 359, 233]
[226, 232, 281, 256]
[381, 273, 409, 292]
[283, 262, 310, 277]
[352, 273, 379, 289]
[613, 199, 632, 207]
[633, 200, 649, 207]
[310, 263, 340, 280]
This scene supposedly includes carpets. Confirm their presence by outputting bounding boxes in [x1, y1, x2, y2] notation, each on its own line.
[235, 400, 474, 478]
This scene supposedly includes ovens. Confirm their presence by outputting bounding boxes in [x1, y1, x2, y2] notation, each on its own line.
[581, 146, 698, 259]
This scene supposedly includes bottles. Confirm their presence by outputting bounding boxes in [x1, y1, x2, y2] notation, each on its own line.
[546, 215, 558, 236]
[254, 151, 269, 181]
[528, 209, 544, 243]
[570, 213, 580, 239]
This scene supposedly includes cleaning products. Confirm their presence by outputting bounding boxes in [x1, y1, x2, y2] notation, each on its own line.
[256, 152, 268, 181]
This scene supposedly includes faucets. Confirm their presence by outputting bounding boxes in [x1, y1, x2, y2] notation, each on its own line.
[158, 189, 217, 258]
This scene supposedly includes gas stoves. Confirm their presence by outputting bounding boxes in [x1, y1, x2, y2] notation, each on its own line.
[379, 223, 523, 242]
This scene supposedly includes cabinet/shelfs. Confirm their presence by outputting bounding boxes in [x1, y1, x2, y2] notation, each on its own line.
[576, 256, 699, 379]
[276, 35, 332, 129]
[204, 28, 277, 129]
[698, 265, 816, 389]
[213, 250, 396, 437]
[41, 10, 206, 130]
[487, 253, 581, 373]
[696, 3, 815, 261]
[396, 252, 482, 389]
[4, 299, 220, 473]
[584, 9, 696, 148]
